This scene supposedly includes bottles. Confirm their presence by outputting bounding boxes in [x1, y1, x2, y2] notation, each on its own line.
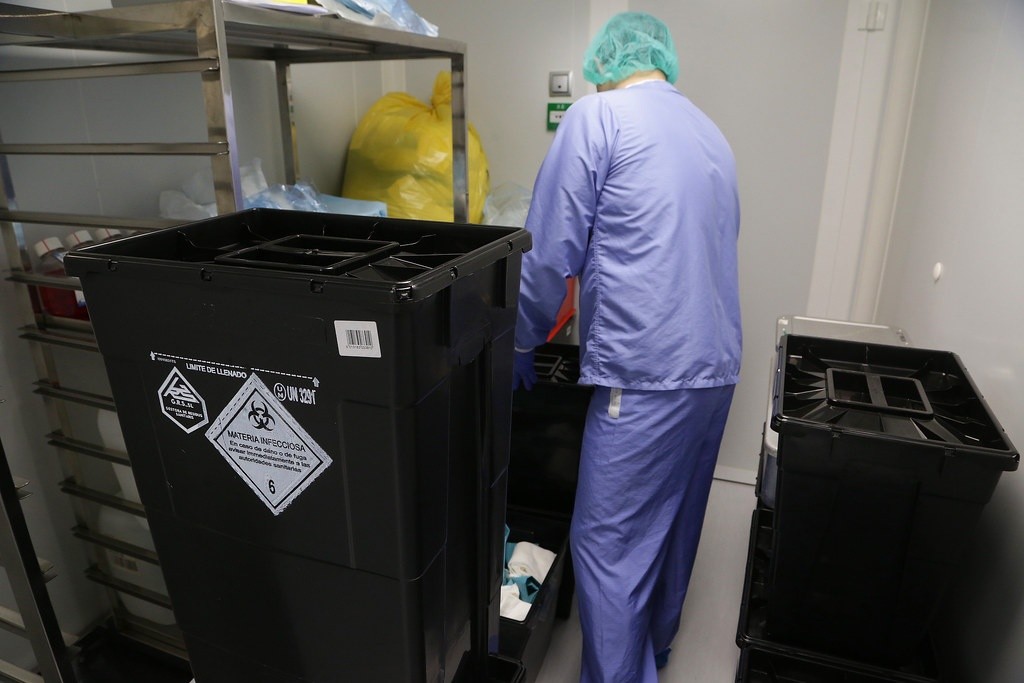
[32, 227, 139, 321]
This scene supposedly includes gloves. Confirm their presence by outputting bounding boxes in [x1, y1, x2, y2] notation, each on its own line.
[512, 349, 537, 391]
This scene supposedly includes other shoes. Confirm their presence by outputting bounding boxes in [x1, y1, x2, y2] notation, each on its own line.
[655, 648, 671, 671]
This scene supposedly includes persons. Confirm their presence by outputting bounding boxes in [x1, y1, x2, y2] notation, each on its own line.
[512, 10, 743, 683]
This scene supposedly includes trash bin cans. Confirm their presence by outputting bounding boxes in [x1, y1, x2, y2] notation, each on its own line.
[734, 330, 1020, 681]
[508, 343, 583, 622]
[64, 205, 533, 683]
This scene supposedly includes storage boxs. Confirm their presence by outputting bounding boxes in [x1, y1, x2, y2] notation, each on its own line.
[61, 206, 590, 683]
[733, 333, 1020, 683]
